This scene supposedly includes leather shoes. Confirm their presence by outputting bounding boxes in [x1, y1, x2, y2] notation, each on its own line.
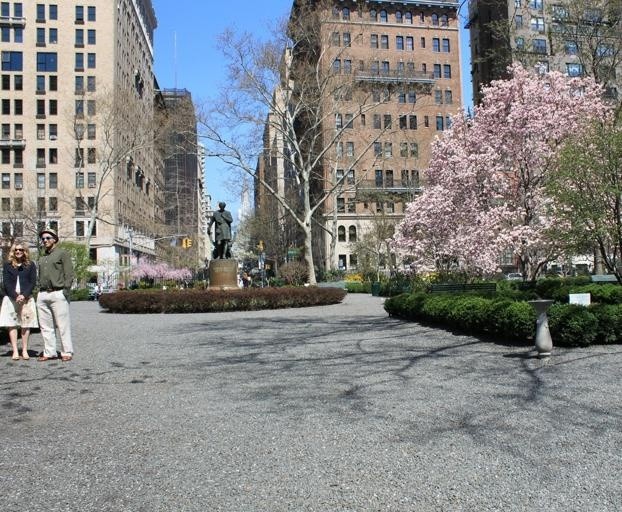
[12, 357, 72, 361]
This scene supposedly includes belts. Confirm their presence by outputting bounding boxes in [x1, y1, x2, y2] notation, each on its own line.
[40, 287, 64, 291]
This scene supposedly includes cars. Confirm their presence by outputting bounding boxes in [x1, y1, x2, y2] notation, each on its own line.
[499, 264, 582, 280]
[73, 284, 117, 299]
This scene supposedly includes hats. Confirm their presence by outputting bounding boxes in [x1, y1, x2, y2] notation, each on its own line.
[39, 229, 58, 243]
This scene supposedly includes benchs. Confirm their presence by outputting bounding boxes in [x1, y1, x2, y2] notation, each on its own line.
[429, 282, 496, 295]
[591, 274, 618, 284]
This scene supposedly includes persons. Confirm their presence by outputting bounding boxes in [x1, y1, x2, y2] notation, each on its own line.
[35, 229, 74, 361]
[207, 202, 233, 260]
[242, 273, 249, 288]
[93, 283, 103, 301]
[0, 241, 37, 360]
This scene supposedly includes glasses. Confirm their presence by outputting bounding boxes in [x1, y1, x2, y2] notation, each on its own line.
[15, 249, 23, 251]
[42, 237, 50, 240]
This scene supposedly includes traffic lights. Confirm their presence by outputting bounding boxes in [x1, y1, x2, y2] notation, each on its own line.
[255, 241, 262, 251]
[182, 238, 192, 248]
[265, 264, 270, 271]
